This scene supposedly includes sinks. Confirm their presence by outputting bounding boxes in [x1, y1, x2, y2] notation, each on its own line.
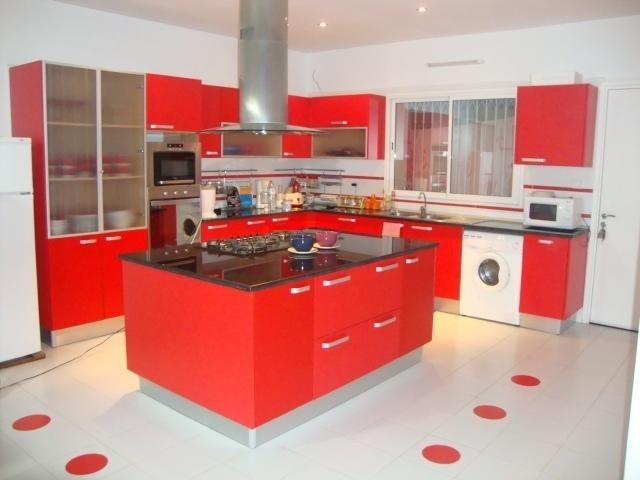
[389, 210, 419, 221]
[415, 212, 451, 223]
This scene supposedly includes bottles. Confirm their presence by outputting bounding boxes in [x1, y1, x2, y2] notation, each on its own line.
[267, 181, 276, 210]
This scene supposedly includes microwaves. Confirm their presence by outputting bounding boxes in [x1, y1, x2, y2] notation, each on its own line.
[523, 196, 582, 230]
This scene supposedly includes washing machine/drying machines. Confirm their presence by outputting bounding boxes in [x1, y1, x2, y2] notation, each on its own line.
[460, 220, 526, 326]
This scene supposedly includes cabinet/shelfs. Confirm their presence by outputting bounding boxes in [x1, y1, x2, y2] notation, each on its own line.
[8, 60, 151, 348]
[199, 85, 240, 158]
[514, 83, 598, 167]
[283, 95, 312, 158]
[148, 75, 201, 134]
[312, 94, 385, 160]
[519, 233, 588, 320]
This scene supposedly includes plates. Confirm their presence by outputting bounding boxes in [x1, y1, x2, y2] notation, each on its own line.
[288, 247, 318, 255]
[50, 210, 133, 236]
[313, 243, 341, 249]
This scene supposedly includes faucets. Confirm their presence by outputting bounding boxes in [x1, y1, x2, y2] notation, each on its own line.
[418, 191, 427, 214]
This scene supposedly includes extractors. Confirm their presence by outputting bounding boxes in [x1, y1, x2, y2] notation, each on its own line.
[196, 0, 329, 135]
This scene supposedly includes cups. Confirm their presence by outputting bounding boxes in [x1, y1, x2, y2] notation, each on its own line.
[316, 232, 338, 247]
[290, 235, 314, 252]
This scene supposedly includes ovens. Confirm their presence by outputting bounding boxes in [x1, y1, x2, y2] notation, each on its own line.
[146, 132, 201, 200]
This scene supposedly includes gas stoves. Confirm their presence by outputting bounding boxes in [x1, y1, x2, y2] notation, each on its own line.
[201, 230, 316, 257]
